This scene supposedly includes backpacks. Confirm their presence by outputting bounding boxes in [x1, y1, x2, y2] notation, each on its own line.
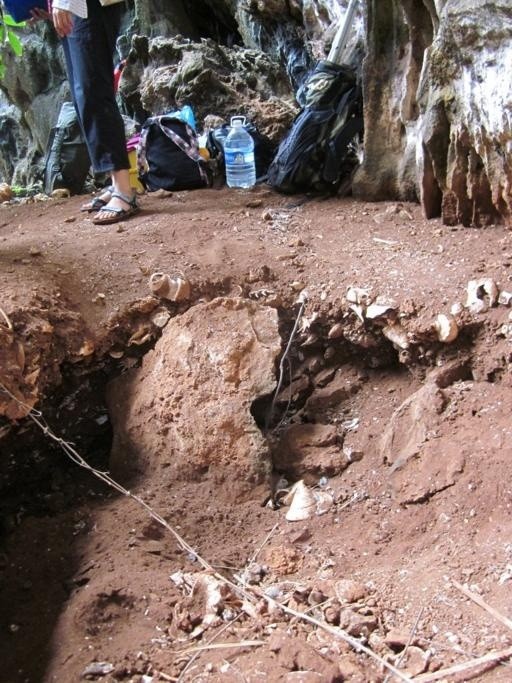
[207, 116, 277, 183]
[268, 59, 362, 200]
[138, 114, 213, 191]
[43, 101, 90, 197]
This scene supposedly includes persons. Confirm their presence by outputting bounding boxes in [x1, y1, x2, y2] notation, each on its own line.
[26, 0, 141, 226]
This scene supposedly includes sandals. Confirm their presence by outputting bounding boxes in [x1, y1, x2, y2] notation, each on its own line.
[92, 191, 142, 224]
[81, 187, 113, 212]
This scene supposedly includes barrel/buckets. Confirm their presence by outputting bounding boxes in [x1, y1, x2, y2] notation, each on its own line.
[224, 114, 256, 189]
